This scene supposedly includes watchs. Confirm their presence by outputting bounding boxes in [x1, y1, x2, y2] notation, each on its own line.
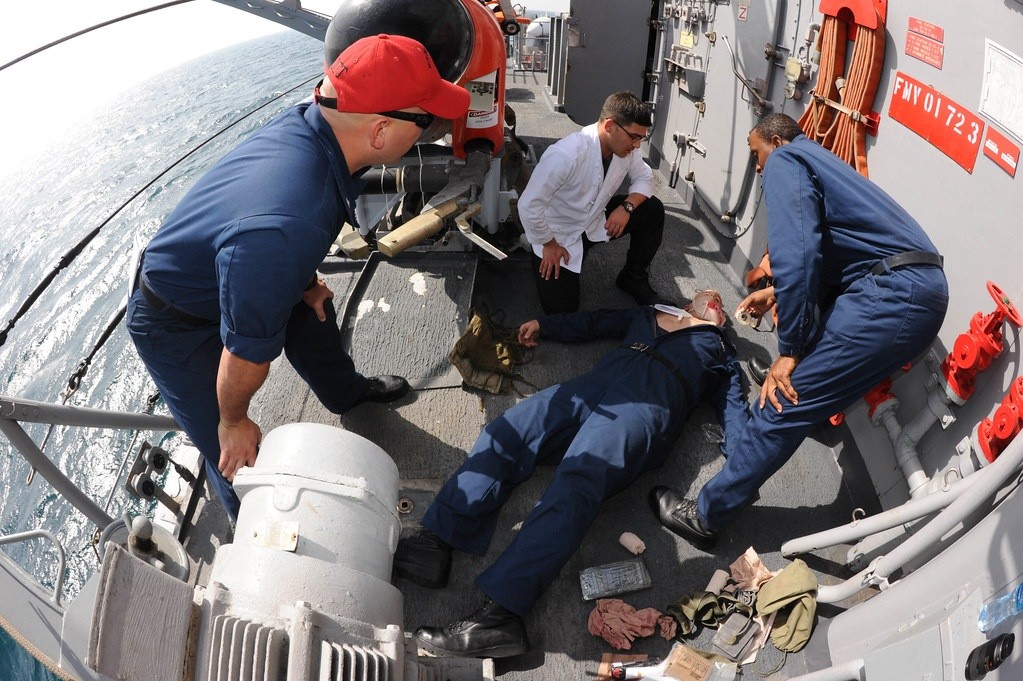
[622, 201, 634, 213]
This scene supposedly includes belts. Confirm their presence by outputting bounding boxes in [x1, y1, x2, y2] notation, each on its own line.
[136, 273, 214, 327]
[623, 341, 694, 406]
[869, 251, 943, 275]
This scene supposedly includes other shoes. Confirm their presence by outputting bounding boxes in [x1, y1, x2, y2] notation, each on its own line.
[615, 268, 661, 307]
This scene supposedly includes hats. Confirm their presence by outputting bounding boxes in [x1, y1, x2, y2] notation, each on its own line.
[327, 33, 471, 119]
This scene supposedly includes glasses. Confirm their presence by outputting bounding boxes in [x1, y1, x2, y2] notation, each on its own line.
[378, 110, 434, 130]
[605, 117, 647, 143]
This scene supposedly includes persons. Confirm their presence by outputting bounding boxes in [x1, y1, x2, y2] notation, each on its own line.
[517, 92, 664, 303]
[125, 36, 470, 529]
[392, 289, 754, 658]
[651, 112, 948, 552]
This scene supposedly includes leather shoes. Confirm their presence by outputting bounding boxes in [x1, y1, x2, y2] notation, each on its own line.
[647, 483, 721, 551]
[747, 356, 773, 387]
[393, 526, 455, 589]
[327, 374, 409, 414]
[413, 594, 530, 658]
